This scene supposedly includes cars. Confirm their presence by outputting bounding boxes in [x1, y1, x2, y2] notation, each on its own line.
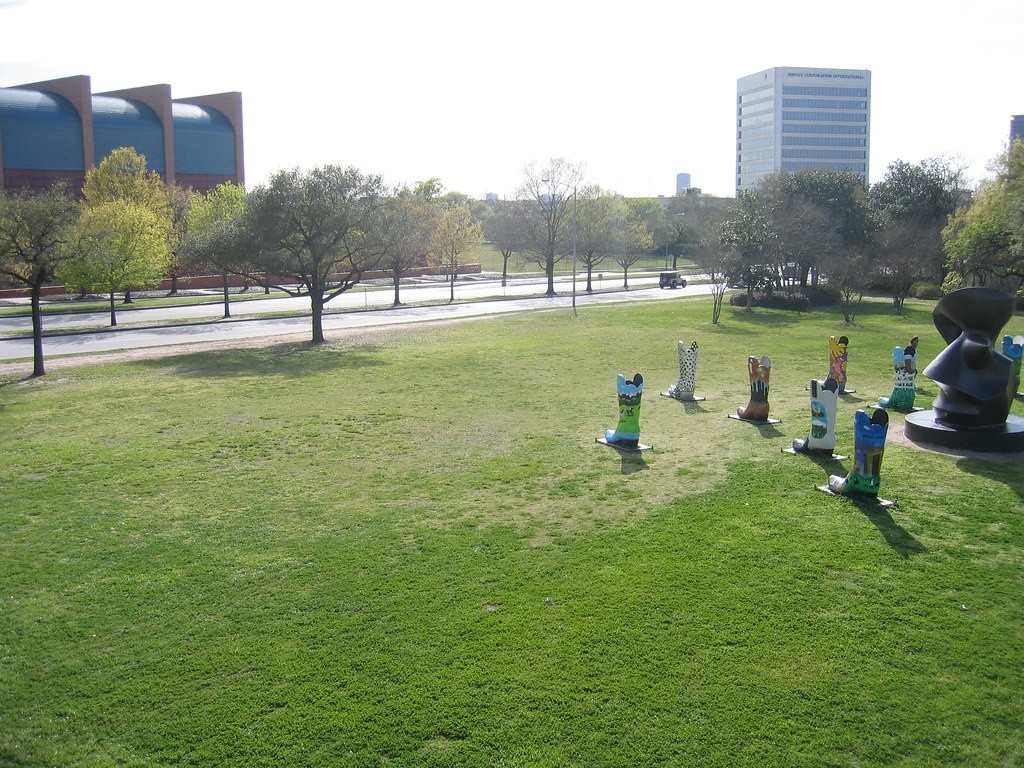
[781, 265, 802, 281]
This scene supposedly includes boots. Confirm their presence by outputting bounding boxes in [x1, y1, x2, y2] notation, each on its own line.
[878, 346, 917, 409]
[605, 373, 643, 447]
[1002, 335, 1024, 395]
[793, 377, 839, 457]
[737, 355, 771, 421]
[828, 408, 888, 504]
[816, 336, 849, 392]
[669, 341, 698, 400]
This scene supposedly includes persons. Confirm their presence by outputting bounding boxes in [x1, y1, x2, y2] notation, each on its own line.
[908, 336, 918, 349]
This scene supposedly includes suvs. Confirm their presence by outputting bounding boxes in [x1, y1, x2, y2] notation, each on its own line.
[658, 271, 686, 290]
